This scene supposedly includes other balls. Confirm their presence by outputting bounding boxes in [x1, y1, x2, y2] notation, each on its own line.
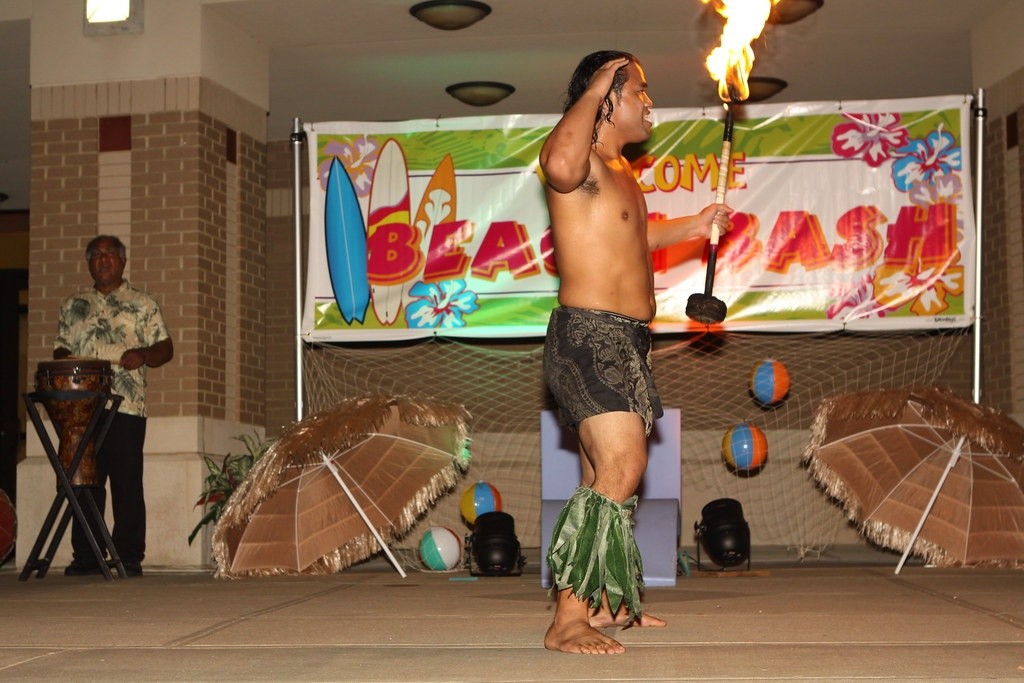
[460, 480, 502, 525]
[418, 525, 462, 572]
[749, 357, 791, 405]
[723, 423, 769, 471]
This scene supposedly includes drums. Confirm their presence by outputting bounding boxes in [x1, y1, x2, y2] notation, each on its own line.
[36, 355, 114, 490]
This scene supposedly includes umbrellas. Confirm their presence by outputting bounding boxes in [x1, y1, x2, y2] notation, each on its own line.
[807, 386, 1024, 575]
[211, 393, 470, 580]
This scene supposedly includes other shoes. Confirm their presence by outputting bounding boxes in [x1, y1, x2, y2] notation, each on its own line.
[64, 559, 104, 575]
[123, 562, 144, 576]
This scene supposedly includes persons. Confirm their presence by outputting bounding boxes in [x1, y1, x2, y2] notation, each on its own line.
[538, 51, 736, 655]
[54, 235, 173, 576]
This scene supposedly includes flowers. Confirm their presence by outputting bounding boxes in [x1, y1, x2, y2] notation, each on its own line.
[188, 429, 277, 546]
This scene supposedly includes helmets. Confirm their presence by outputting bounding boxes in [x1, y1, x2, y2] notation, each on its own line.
[471, 511, 519, 574]
[696, 497, 750, 567]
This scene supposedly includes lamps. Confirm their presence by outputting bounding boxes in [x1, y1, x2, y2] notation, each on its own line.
[766, 0, 825, 25]
[445, 81, 516, 107]
[694, 498, 751, 573]
[464, 512, 527, 578]
[83, 0, 144, 36]
[409, 0, 492, 32]
[748, 76, 787, 103]
